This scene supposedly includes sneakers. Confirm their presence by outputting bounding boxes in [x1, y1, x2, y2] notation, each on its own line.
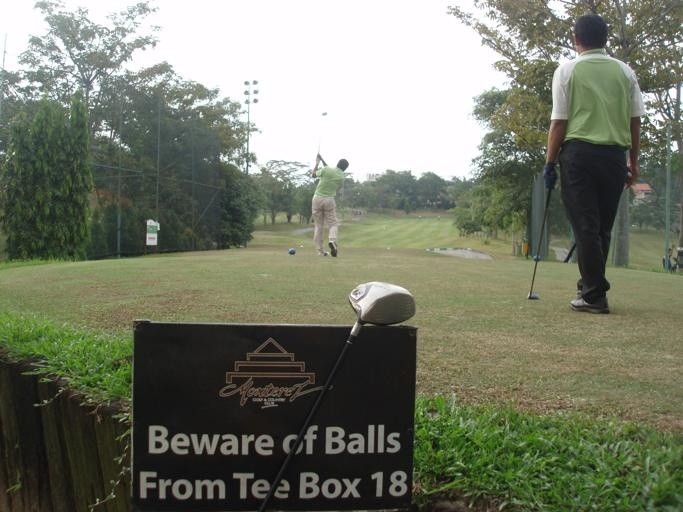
[329, 241, 337, 257]
[570, 288, 609, 314]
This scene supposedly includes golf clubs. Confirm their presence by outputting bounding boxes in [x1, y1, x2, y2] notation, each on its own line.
[317, 112, 326, 155]
[261, 281, 415, 510]
[527, 188, 553, 300]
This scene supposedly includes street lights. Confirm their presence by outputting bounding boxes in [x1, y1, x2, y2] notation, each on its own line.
[242, 79, 259, 174]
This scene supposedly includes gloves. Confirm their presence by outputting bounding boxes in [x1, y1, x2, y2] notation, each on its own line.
[543, 162, 556, 189]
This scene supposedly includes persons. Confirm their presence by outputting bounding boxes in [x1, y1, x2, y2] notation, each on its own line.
[662, 248, 677, 273]
[352, 207, 361, 217]
[311, 153, 349, 256]
[543, 14, 644, 315]
